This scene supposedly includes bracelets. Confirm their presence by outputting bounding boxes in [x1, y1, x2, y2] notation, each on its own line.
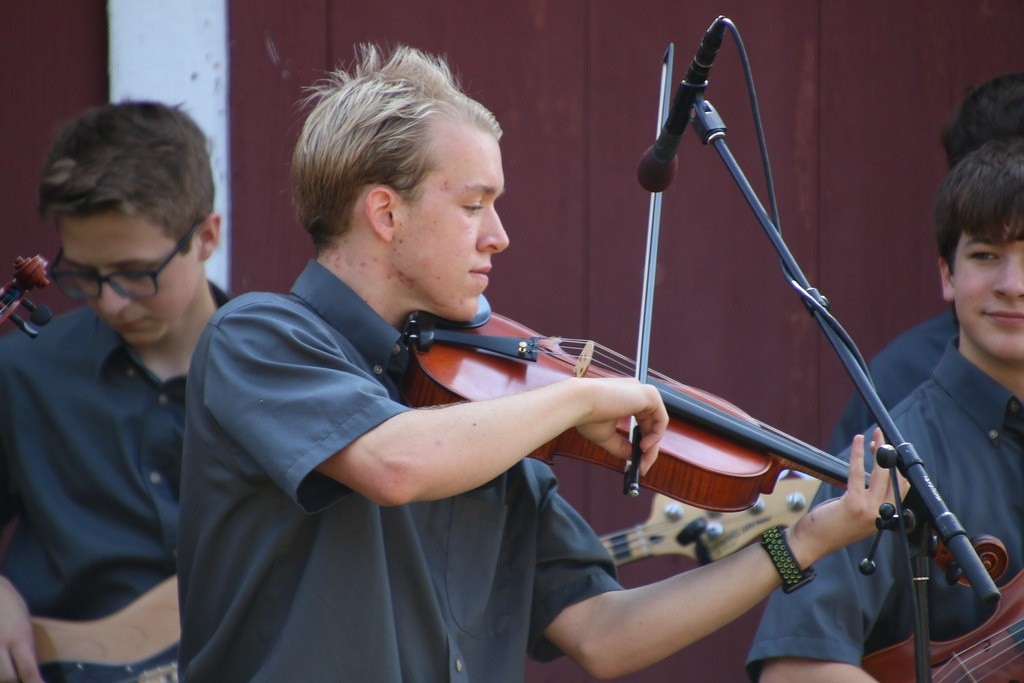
[759, 525, 816, 594]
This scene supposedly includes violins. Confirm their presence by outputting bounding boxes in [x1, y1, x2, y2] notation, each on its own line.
[0, 254, 50, 338]
[862, 568, 1024, 683]
[401, 294, 1009, 590]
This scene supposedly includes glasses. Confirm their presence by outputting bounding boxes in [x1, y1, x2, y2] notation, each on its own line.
[49, 221, 203, 302]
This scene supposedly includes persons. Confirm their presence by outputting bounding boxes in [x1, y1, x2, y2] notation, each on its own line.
[0, 99, 231, 683]
[177, 42, 913, 683]
[743, 138, 1024, 683]
[828, 74, 1024, 457]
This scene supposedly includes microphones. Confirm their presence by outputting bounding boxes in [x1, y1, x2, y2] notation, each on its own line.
[636, 15, 727, 192]
